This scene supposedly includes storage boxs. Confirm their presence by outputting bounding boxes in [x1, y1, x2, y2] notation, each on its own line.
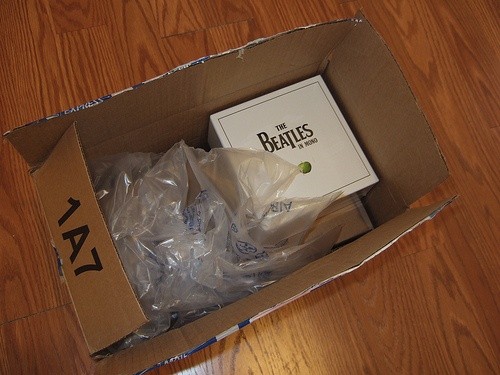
[2, 9, 461, 375]
